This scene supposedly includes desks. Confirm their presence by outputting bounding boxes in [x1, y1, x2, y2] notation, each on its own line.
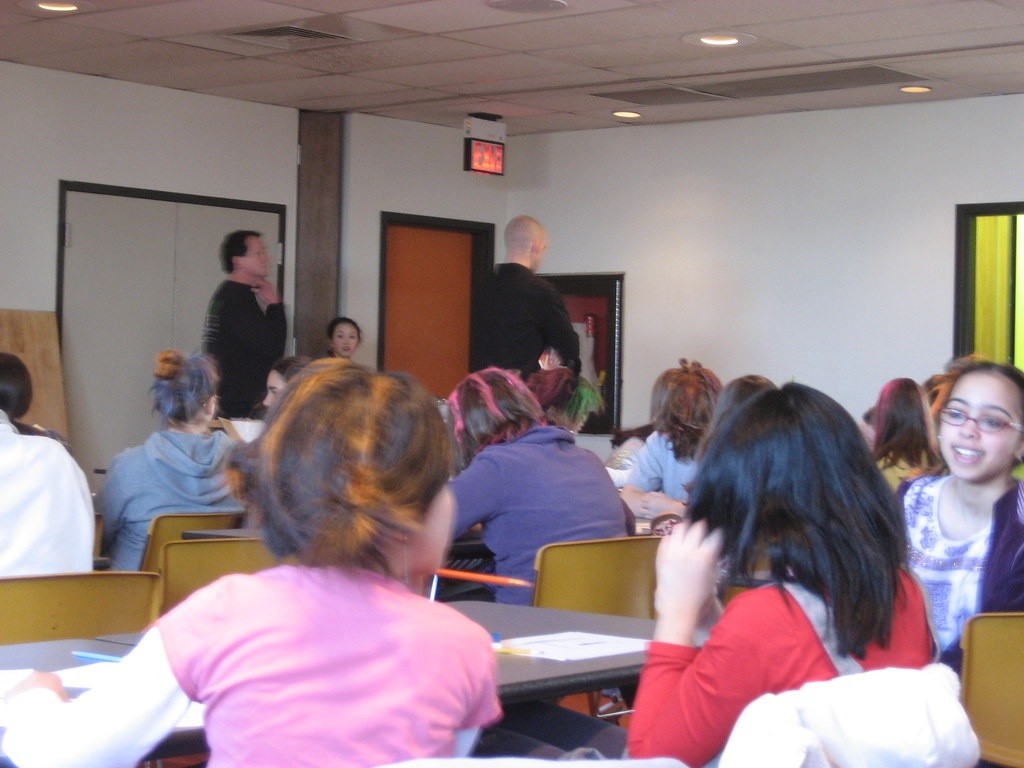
[0, 600, 654, 707]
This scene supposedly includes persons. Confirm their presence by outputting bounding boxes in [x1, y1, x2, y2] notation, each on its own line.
[474, 216, 582, 383]
[101, 316, 948, 720]
[628, 381, 933, 768]
[0, 357, 504, 768]
[201, 231, 289, 419]
[894, 354, 1024, 675]
[0, 351, 96, 579]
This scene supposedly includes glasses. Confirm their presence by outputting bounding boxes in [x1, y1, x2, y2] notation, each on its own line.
[937, 407, 1024, 433]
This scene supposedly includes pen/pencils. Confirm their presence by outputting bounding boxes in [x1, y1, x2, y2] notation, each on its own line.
[71, 651, 122, 662]
[435, 568, 535, 588]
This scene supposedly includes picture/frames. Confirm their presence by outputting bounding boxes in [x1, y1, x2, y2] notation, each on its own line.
[537, 271, 621, 435]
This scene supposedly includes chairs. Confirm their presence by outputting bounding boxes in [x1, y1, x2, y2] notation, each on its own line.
[425, 535, 658, 619]
[958, 612, 1023, 768]
[159, 537, 273, 616]
[0, 570, 161, 646]
[139, 511, 246, 572]
